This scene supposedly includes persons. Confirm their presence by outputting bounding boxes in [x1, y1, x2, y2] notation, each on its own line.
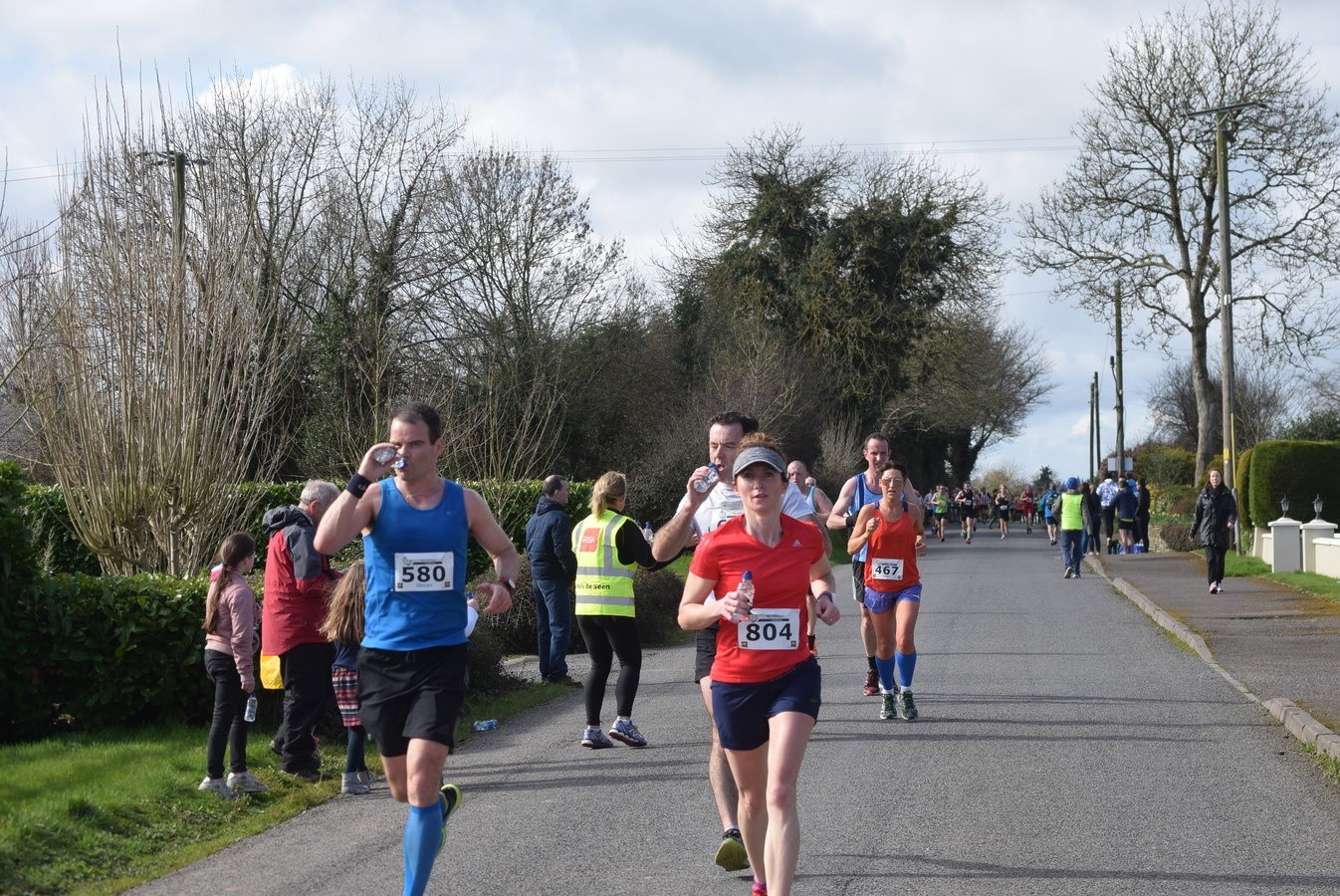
[846, 462, 929, 721]
[464, 592, 479, 638]
[1050, 477, 1093, 580]
[649, 412, 833, 873]
[1187, 468, 1238, 595]
[826, 433, 925, 695]
[329, 559, 378, 794]
[910, 476, 1154, 557]
[260, 480, 341, 783]
[314, 400, 521, 896]
[197, 532, 270, 799]
[803, 477, 818, 487]
[787, 460, 836, 657]
[526, 474, 584, 687]
[675, 433, 840, 896]
[568, 472, 699, 749]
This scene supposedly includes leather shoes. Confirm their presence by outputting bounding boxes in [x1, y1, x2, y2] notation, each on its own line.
[550, 677, 583, 688]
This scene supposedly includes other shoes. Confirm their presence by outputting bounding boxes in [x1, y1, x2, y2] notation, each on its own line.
[1005, 531, 1008, 534]
[1051, 540, 1054, 545]
[937, 532, 940, 538]
[973, 526, 976, 531]
[1027, 528, 1029, 533]
[1090, 551, 1094, 555]
[1029, 528, 1032, 533]
[1054, 541, 1057, 544]
[935, 528, 938, 533]
[1001, 534, 1006, 539]
[1096, 552, 1100, 556]
[966, 538, 970, 544]
[940, 537, 944, 541]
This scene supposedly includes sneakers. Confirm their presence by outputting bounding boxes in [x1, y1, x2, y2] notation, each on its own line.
[580, 727, 615, 749]
[431, 784, 460, 854]
[1120, 551, 1126, 555]
[1064, 566, 1072, 579]
[751, 881, 766, 895]
[270, 740, 282, 756]
[1073, 574, 1081, 578]
[226, 772, 269, 793]
[714, 829, 750, 872]
[282, 767, 333, 786]
[1217, 582, 1221, 592]
[1208, 581, 1217, 593]
[1107, 537, 1112, 548]
[609, 720, 647, 746]
[197, 776, 234, 798]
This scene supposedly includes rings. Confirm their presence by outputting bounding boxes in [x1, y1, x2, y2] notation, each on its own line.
[924, 551, 926, 555]
[829, 617, 833, 623]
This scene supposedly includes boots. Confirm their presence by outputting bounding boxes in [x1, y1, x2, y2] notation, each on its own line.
[359, 769, 376, 784]
[339, 771, 370, 795]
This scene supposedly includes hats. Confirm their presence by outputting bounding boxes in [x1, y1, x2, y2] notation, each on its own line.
[732, 447, 788, 477]
[1067, 477, 1078, 489]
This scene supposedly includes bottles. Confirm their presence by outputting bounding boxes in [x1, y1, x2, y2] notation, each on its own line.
[695, 463, 723, 493]
[734, 570, 754, 620]
[374, 445, 408, 473]
[643, 521, 657, 547]
[475, 719, 501, 734]
[245, 693, 258, 722]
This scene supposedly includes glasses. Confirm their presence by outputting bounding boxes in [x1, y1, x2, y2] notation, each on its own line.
[881, 477, 904, 487]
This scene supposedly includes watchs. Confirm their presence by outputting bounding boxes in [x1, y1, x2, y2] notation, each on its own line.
[498, 575, 518, 597]
[816, 591, 837, 604]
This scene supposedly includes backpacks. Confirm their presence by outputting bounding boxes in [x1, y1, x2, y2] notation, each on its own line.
[1045, 492, 1058, 509]
